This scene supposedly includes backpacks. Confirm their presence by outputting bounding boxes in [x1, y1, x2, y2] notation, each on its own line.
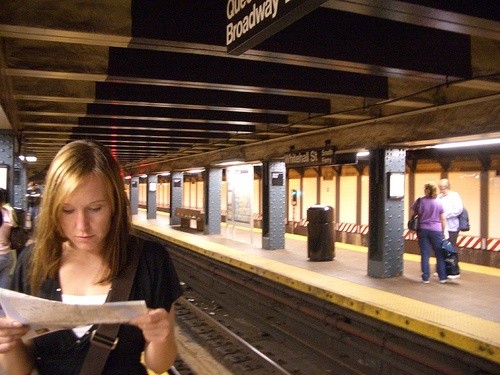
[8, 214, 27, 248]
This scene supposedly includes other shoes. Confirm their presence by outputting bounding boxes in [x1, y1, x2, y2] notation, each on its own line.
[433, 272, 438, 276]
[424, 280, 430, 283]
[440, 279, 447, 283]
[447, 274, 460, 279]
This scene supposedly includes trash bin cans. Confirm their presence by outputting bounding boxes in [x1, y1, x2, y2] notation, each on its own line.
[306, 207, 336, 261]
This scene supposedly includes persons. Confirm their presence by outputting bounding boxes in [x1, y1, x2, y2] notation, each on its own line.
[0, 188, 17, 316]
[27, 182, 42, 228]
[434, 179, 463, 278]
[410, 183, 451, 283]
[0, 141, 184, 375]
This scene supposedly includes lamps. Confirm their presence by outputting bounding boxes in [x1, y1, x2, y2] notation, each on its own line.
[18, 151, 37, 162]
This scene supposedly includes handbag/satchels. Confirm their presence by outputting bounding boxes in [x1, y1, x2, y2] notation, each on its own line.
[458, 207, 470, 231]
[441, 238, 460, 275]
[408, 197, 420, 231]
